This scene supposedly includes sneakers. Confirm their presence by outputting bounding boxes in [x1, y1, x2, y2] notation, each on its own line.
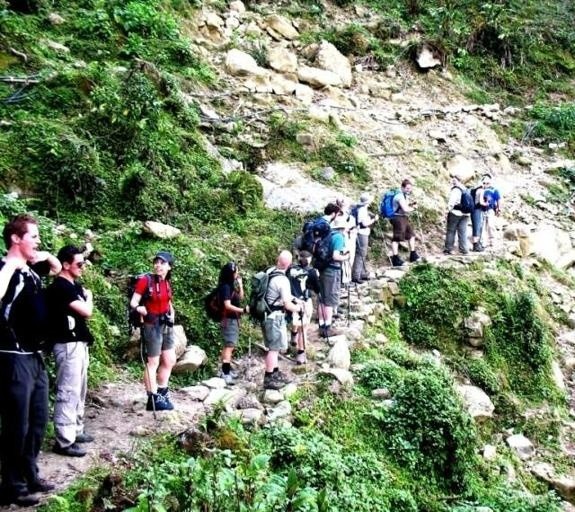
[147, 393, 167, 410]
[75, 433, 93, 443]
[319, 272, 371, 337]
[220, 371, 238, 380]
[158, 387, 173, 410]
[53, 441, 86, 457]
[222, 374, 236, 386]
[442, 237, 496, 255]
[297, 351, 307, 365]
[392, 254, 404, 266]
[409, 250, 418, 262]
[274, 370, 292, 384]
[289, 331, 298, 347]
[263, 374, 285, 389]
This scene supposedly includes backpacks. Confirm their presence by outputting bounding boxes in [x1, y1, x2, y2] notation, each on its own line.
[380, 191, 400, 219]
[471, 185, 486, 210]
[311, 234, 335, 269]
[300, 220, 328, 252]
[248, 268, 286, 322]
[205, 288, 225, 322]
[452, 186, 475, 213]
[350, 204, 361, 226]
[125, 272, 152, 328]
[288, 269, 310, 301]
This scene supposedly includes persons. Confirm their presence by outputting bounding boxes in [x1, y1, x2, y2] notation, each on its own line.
[0, 214, 62, 506]
[48, 243, 93, 458]
[128, 252, 177, 409]
[303, 179, 422, 338]
[443, 174, 500, 254]
[215, 251, 319, 391]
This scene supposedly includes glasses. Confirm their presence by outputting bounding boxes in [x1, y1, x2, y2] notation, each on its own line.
[77, 260, 86, 269]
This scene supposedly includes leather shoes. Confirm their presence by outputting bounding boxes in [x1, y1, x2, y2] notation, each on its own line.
[26, 476, 56, 491]
[0, 490, 38, 506]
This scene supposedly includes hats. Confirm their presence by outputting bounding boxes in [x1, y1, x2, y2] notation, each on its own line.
[331, 218, 347, 230]
[357, 193, 374, 208]
[154, 252, 174, 265]
